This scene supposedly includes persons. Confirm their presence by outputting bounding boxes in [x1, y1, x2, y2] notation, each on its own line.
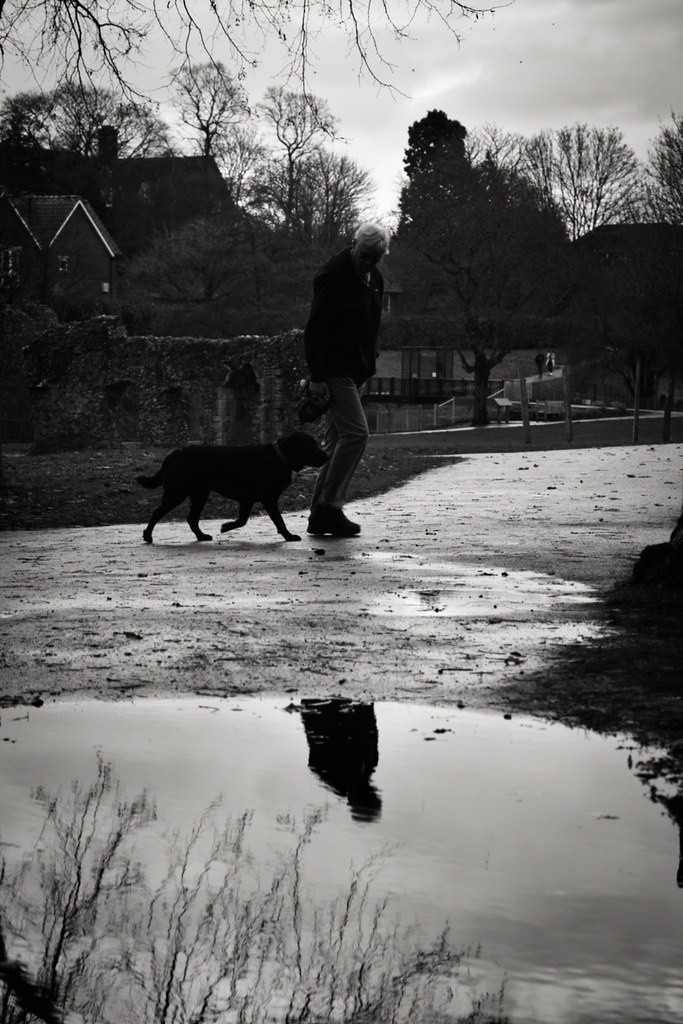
[296, 697, 385, 824]
[545, 348, 556, 376]
[536, 351, 546, 379]
[302, 223, 389, 538]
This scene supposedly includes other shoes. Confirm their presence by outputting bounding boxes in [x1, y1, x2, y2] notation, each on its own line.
[306, 501, 362, 537]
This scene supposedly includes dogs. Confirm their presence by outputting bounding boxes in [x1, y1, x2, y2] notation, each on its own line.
[134, 430, 330, 546]
